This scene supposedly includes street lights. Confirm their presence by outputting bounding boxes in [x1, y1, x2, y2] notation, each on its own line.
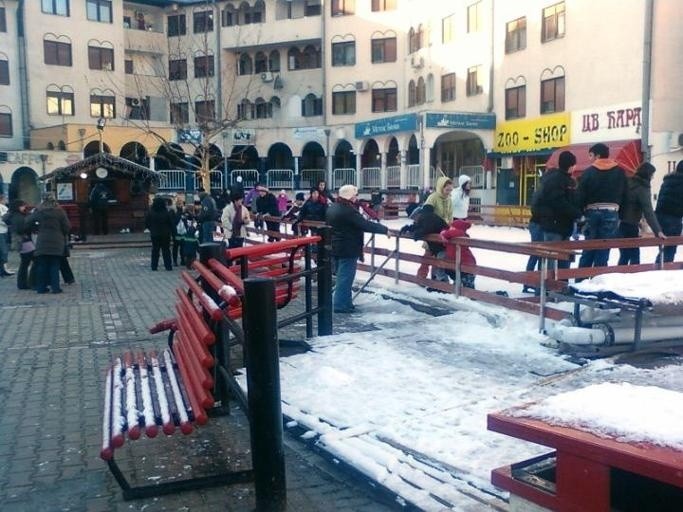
[96, 115, 107, 151]
[39, 153, 47, 198]
[220, 132, 228, 187]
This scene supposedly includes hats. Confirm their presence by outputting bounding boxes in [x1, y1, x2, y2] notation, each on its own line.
[452, 220, 467, 232]
[406, 202, 420, 219]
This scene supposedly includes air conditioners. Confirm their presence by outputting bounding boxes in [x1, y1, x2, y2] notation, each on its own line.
[669, 131, 683, 149]
[356, 81, 368, 92]
[260, 72, 273, 82]
[132, 97, 143, 107]
[410, 55, 422, 67]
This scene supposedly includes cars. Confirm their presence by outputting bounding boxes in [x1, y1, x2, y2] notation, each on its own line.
[154, 192, 178, 211]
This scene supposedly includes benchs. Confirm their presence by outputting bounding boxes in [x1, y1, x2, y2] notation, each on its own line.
[100, 259, 254, 501]
[150, 236, 322, 356]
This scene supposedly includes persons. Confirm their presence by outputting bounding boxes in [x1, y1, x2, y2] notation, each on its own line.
[522, 144, 682, 297]
[0, 190, 75, 293]
[146, 175, 477, 314]
[89, 182, 113, 236]
